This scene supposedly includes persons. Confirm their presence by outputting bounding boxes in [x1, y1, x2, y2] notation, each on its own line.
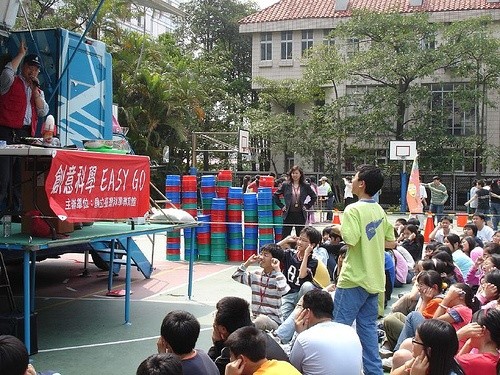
[157, 309, 221, 375]
[232, 244, 290, 330]
[342, 176, 354, 210]
[379, 212, 500, 375]
[225, 326, 302, 375]
[312, 218, 424, 333]
[208, 297, 292, 375]
[330, 164, 395, 375]
[467, 180, 484, 224]
[0, 40, 49, 222]
[136, 353, 183, 375]
[264, 226, 322, 344]
[289, 289, 364, 375]
[274, 166, 317, 240]
[419, 176, 448, 242]
[0, 335, 62, 375]
[242, 172, 333, 223]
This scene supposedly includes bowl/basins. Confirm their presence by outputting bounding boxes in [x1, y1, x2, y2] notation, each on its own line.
[81, 140, 116, 149]
[23, 137, 44, 145]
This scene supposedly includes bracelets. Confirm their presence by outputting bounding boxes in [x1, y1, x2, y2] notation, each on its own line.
[439, 303, 448, 309]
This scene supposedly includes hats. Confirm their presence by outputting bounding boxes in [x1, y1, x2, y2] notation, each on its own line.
[331, 227, 341, 236]
[318, 176, 328, 182]
[24, 54, 41, 64]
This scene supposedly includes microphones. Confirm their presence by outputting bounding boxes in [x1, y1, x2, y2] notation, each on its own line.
[29, 74, 41, 88]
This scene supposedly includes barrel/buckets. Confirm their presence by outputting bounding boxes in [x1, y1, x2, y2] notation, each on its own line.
[457, 213, 468, 227]
[211, 198, 226, 264]
[244, 193, 258, 262]
[196, 215, 211, 261]
[272, 187, 285, 243]
[182, 175, 198, 261]
[258, 176, 275, 254]
[201, 175, 216, 215]
[227, 187, 243, 261]
[166, 175, 180, 261]
[216, 170, 231, 198]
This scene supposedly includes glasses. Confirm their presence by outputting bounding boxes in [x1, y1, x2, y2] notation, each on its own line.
[24, 64, 40, 73]
[411, 336, 424, 346]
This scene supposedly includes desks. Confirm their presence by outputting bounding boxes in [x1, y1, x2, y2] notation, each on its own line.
[0, 148, 135, 240]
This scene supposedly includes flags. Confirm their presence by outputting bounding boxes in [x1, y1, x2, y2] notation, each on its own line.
[407, 156, 423, 213]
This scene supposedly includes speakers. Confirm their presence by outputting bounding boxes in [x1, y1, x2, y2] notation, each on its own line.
[0, 310, 38, 356]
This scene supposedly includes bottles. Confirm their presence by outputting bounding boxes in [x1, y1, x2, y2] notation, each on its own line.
[3, 215, 11, 237]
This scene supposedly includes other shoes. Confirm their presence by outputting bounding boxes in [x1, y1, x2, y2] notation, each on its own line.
[265, 329, 281, 344]
[379, 348, 393, 358]
[326, 220, 331, 222]
[10, 214, 21, 223]
[382, 357, 392, 369]
[377, 328, 386, 339]
[376, 321, 385, 330]
[398, 291, 409, 298]
